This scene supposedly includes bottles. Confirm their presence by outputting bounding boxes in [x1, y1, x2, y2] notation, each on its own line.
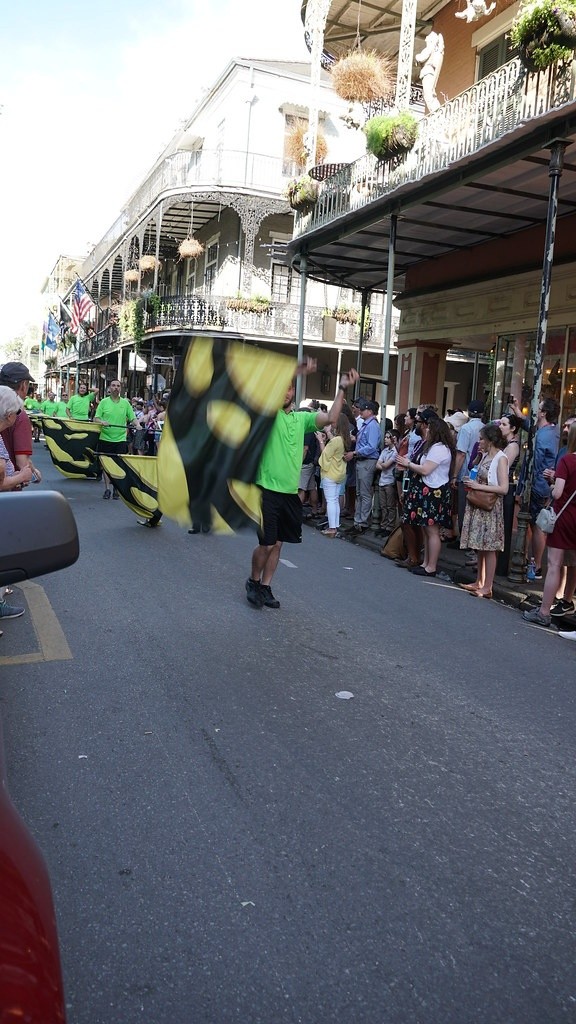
[468, 465, 479, 481]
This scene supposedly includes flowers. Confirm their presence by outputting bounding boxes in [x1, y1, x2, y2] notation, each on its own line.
[503, 0, 576, 67]
[229, 295, 270, 315]
[322, 307, 362, 325]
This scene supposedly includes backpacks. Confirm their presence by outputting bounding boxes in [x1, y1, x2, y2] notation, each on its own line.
[380, 525, 406, 560]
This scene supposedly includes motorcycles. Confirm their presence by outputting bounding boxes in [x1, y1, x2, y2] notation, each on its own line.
[22, 404, 43, 441]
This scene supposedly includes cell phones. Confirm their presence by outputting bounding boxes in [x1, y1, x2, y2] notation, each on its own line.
[402, 478, 410, 492]
[510, 395, 514, 405]
[351, 428, 359, 436]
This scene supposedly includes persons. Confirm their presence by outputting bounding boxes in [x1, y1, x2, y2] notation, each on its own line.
[53, 393, 70, 419]
[66, 383, 99, 420]
[243, 356, 360, 609]
[23, 392, 44, 411]
[41, 393, 58, 417]
[0, 361, 41, 595]
[0, 385, 27, 634]
[188, 523, 211, 534]
[415, 30, 445, 113]
[126, 394, 169, 456]
[0, 780, 67, 1024]
[94, 379, 144, 501]
[290, 396, 576, 640]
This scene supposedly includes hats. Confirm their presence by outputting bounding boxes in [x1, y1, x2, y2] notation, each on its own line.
[418, 409, 438, 423]
[444, 411, 468, 431]
[0, 362, 35, 383]
[468, 399, 484, 413]
[356, 400, 378, 415]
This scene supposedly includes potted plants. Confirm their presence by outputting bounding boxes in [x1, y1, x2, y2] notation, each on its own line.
[365, 111, 416, 159]
[133, 295, 162, 350]
[284, 180, 317, 210]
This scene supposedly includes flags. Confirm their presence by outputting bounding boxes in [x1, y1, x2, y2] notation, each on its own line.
[41, 324, 49, 351]
[41, 420, 101, 480]
[45, 315, 61, 351]
[58, 303, 72, 350]
[68, 279, 95, 334]
[99, 453, 160, 520]
[29, 414, 64, 429]
[154, 335, 299, 535]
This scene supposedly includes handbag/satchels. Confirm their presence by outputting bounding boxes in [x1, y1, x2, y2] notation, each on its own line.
[535, 506, 556, 534]
[466, 489, 498, 511]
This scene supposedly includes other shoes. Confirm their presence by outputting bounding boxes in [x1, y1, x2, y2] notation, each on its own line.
[113, 492, 119, 499]
[470, 590, 492, 598]
[202, 523, 210, 532]
[535, 568, 542, 579]
[103, 490, 111, 499]
[458, 583, 479, 591]
[303, 503, 389, 538]
[188, 527, 199, 534]
[393, 531, 478, 575]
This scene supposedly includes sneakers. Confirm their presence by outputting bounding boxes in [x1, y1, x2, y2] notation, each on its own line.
[523, 608, 552, 627]
[262, 586, 281, 608]
[246, 578, 264, 607]
[550, 600, 574, 617]
[0, 600, 25, 619]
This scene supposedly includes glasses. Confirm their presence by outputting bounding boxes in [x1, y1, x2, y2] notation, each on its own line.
[16, 409, 21, 415]
[502, 413, 511, 418]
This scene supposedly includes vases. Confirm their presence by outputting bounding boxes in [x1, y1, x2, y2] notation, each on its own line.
[515, 13, 576, 71]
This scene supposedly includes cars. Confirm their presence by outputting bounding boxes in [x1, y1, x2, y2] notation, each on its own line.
[0, 490, 80, 1024]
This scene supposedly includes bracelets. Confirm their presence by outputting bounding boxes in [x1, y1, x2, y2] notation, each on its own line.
[339, 385, 345, 392]
